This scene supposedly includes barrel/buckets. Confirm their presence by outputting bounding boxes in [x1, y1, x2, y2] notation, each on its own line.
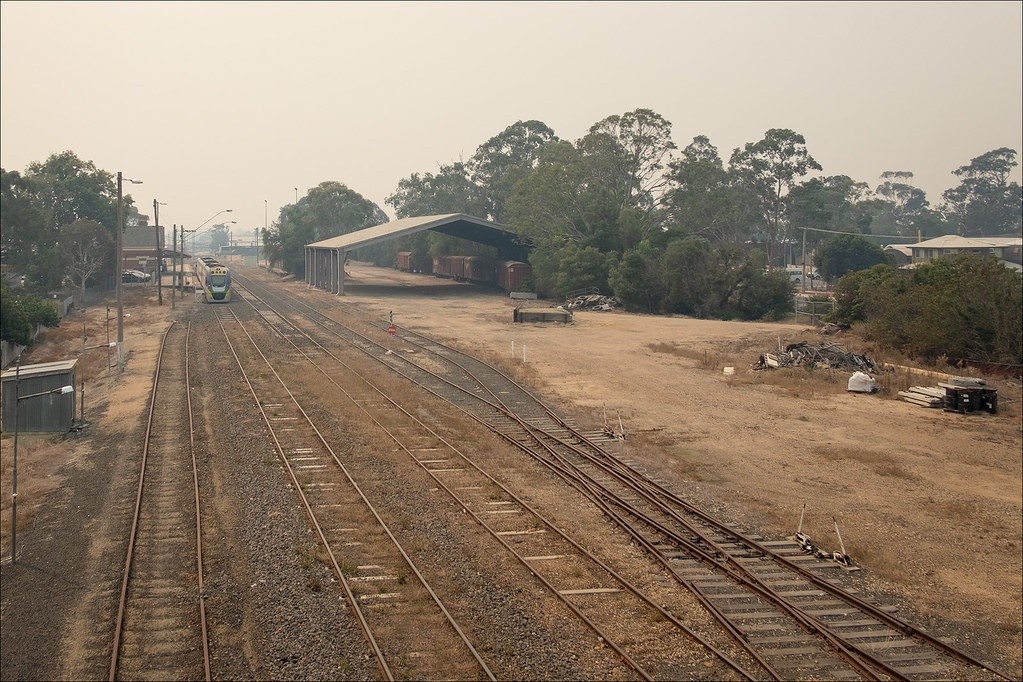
[945, 387, 998, 415]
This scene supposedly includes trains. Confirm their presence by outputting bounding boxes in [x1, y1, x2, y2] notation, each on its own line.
[196, 257, 232, 303]
[397, 252, 532, 293]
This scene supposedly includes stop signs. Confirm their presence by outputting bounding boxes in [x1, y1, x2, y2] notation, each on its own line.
[388, 327, 396, 333]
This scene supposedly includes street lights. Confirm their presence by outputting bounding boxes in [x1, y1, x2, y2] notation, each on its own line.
[180, 221, 238, 297]
[12, 386, 74, 563]
[153, 199, 167, 306]
[173, 209, 232, 308]
[295, 188, 297, 203]
[81, 343, 117, 424]
[117, 172, 143, 362]
[107, 314, 131, 370]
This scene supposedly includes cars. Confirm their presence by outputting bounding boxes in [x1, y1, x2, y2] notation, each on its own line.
[215, 246, 231, 255]
[122, 273, 143, 283]
[125, 271, 151, 281]
[807, 268, 823, 280]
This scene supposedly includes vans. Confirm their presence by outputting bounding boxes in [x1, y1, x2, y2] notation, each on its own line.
[785, 268, 803, 283]
